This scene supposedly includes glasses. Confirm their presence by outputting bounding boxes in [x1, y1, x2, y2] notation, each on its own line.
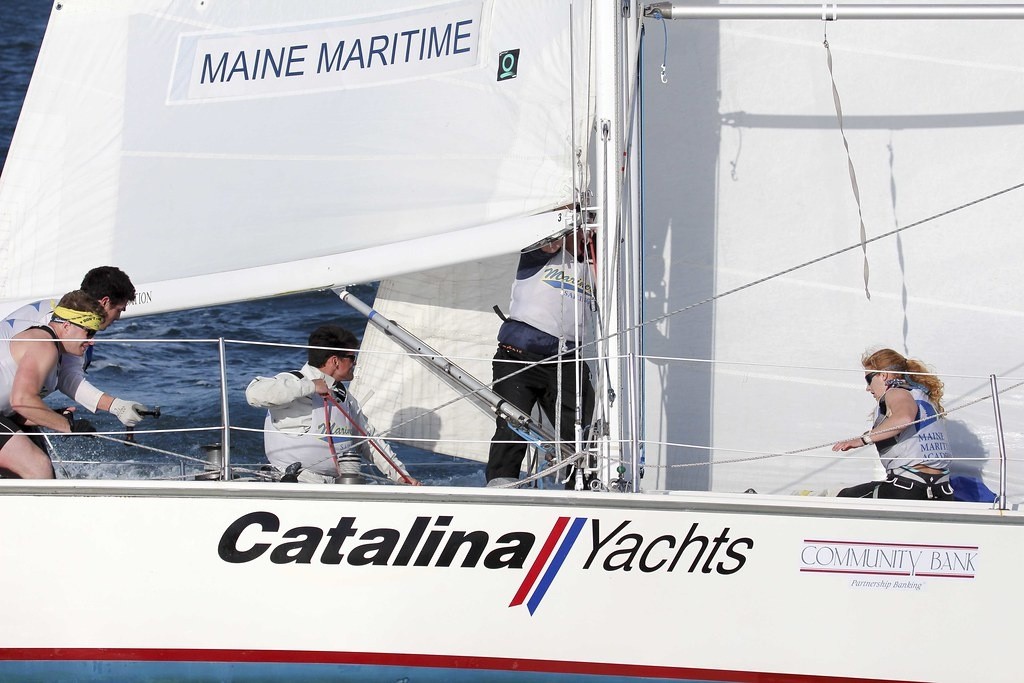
[68, 320, 97, 339]
[336, 354, 356, 361]
[865, 372, 878, 386]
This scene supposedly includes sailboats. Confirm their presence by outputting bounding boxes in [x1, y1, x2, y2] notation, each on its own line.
[0, 0, 1024, 683]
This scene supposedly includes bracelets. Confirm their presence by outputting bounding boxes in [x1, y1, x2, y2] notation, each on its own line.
[861, 437, 867, 445]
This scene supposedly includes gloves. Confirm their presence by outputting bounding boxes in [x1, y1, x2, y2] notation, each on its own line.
[68, 414, 97, 438]
[107, 397, 148, 427]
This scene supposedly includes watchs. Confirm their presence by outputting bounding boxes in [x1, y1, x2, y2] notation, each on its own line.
[862, 430, 874, 445]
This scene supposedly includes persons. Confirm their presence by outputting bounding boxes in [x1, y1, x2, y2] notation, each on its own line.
[245, 325, 425, 485]
[832, 348, 954, 501]
[484, 201, 600, 490]
[0, 265, 147, 479]
